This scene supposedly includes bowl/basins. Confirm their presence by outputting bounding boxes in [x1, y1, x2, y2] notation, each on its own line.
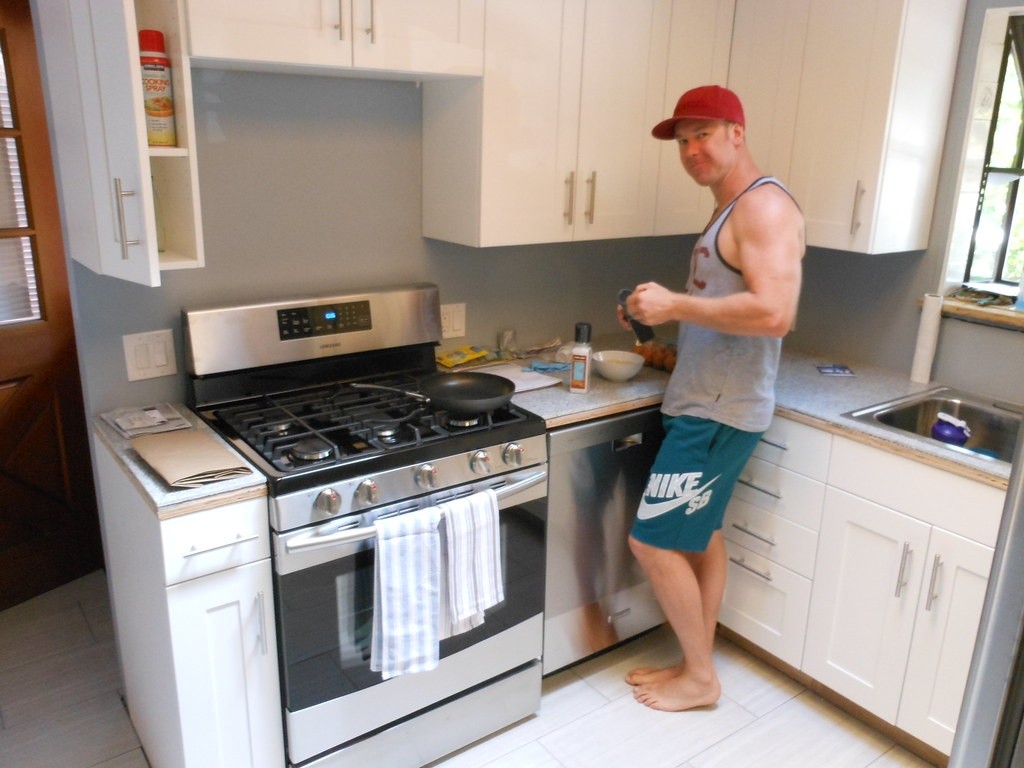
[592, 350, 646, 383]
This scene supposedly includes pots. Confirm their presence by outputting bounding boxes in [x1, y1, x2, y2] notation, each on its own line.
[349, 371, 515, 417]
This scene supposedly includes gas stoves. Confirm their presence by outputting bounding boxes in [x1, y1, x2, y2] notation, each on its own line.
[181, 282, 547, 499]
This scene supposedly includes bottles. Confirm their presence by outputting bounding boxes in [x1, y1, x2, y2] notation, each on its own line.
[569, 323, 593, 395]
[138, 29, 178, 149]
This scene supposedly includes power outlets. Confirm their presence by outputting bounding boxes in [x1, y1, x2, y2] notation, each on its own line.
[440, 302, 465, 339]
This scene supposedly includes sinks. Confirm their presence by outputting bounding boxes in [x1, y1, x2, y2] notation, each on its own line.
[872, 397, 1024, 465]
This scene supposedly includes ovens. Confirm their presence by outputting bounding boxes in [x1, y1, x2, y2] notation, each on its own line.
[270, 433, 550, 768]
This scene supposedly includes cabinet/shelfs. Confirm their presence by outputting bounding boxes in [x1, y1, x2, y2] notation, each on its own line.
[715, 414, 1008, 768]
[37, 0, 966, 289]
[93, 434, 287, 768]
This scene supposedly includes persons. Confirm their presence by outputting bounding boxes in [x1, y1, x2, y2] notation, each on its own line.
[619, 86, 807, 711]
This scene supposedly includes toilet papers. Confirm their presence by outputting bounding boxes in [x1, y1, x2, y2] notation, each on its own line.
[909, 293, 944, 385]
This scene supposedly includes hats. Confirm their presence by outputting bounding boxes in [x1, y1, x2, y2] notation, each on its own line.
[652, 86, 746, 141]
